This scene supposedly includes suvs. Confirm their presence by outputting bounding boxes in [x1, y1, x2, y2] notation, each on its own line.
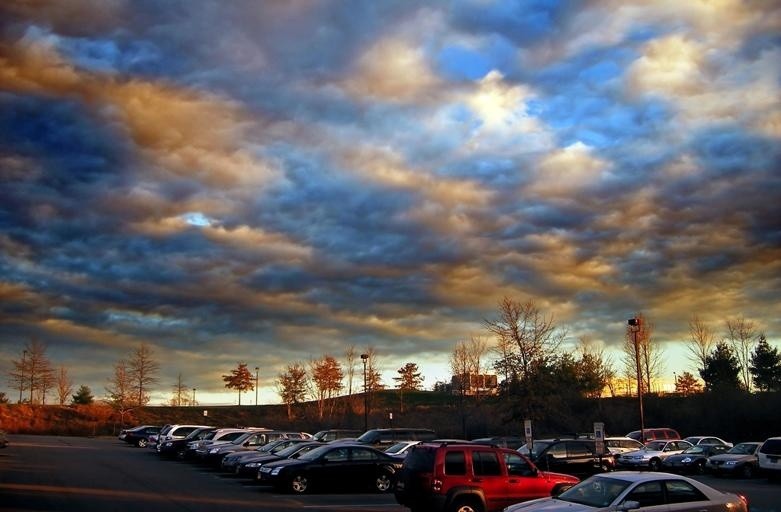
[393, 439, 581, 512]
[505, 439, 616, 479]
[148, 423, 314, 465]
[756, 437, 780, 471]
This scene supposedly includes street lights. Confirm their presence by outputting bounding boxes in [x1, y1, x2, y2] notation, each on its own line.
[627, 316, 645, 444]
[19, 350, 27, 403]
[192, 388, 196, 407]
[253, 367, 259, 406]
[360, 354, 369, 433]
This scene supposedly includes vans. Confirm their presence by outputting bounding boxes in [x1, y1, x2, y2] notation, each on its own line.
[355, 428, 438, 446]
[625, 428, 681, 445]
[603, 437, 646, 467]
[472, 437, 522, 450]
[309, 429, 362, 443]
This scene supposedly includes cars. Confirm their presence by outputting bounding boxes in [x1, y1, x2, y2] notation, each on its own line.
[117, 425, 160, 448]
[501, 471, 749, 512]
[221, 437, 422, 497]
[618, 435, 762, 479]
